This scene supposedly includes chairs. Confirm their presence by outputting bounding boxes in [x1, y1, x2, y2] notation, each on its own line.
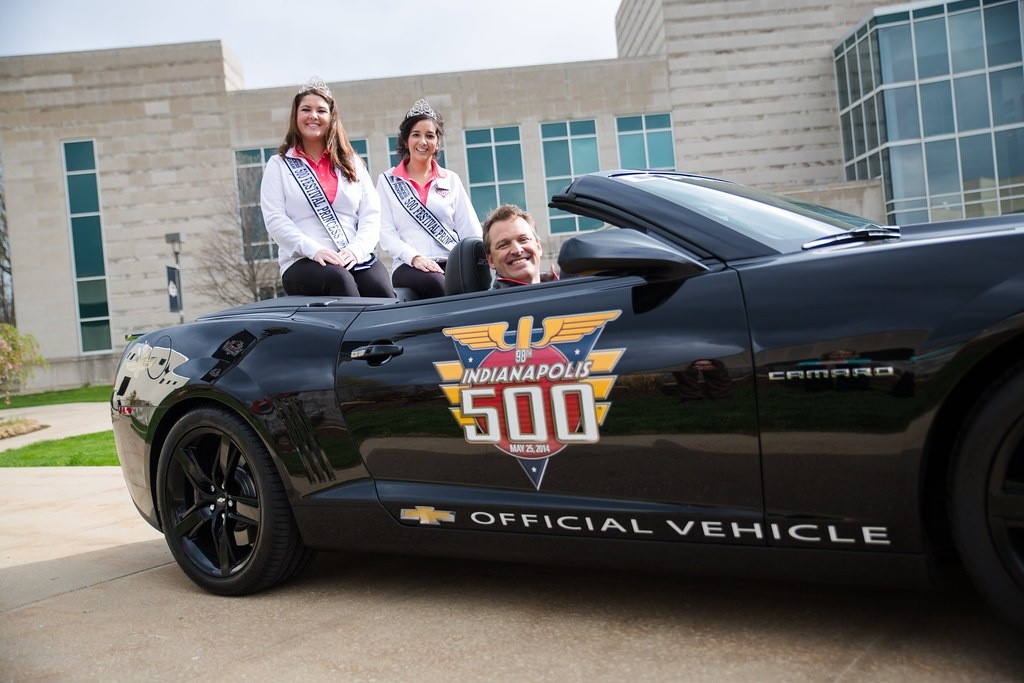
[445, 235, 493, 298]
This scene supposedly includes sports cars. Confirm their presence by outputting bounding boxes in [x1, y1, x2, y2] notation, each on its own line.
[110, 169, 1022, 632]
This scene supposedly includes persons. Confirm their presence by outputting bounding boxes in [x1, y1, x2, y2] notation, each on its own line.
[484, 203, 559, 290]
[377, 98, 483, 301]
[690, 359, 724, 370]
[260, 75, 398, 299]
[822, 349, 855, 360]
